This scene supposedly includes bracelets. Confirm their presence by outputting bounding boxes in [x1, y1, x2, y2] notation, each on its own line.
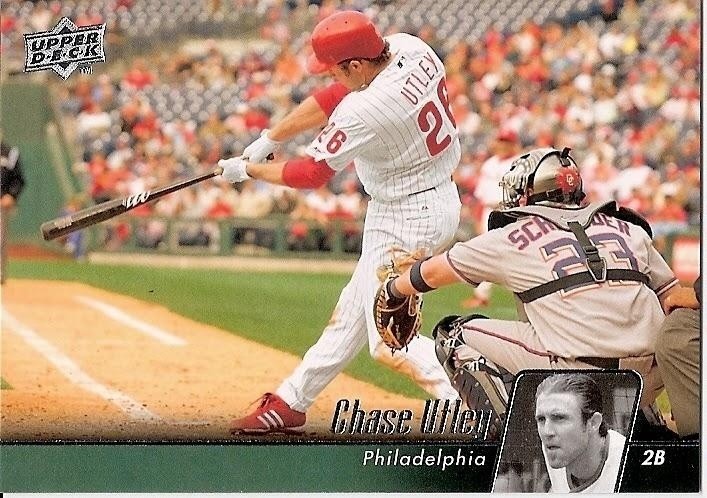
[406, 255, 439, 294]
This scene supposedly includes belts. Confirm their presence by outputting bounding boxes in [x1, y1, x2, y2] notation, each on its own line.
[576, 353, 656, 371]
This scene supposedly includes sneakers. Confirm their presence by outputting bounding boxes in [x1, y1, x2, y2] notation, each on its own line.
[228, 393, 305, 435]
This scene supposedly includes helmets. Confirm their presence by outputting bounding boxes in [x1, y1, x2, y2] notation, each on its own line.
[306, 11, 385, 73]
[500, 147, 586, 210]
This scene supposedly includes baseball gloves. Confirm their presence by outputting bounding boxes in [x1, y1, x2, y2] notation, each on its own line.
[373, 263, 423, 350]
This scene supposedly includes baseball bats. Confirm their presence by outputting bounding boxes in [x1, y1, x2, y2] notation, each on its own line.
[40, 150, 273, 242]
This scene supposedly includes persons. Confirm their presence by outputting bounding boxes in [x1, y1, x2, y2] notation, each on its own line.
[371, 144, 688, 436]
[1, 138, 27, 283]
[655, 270, 702, 438]
[39, 9, 463, 425]
[531, 370, 626, 491]
[0, 0, 703, 260]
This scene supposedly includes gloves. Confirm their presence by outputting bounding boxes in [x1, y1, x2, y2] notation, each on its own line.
[242, 128, 284, 164]
[218, 157, 250, 183]
